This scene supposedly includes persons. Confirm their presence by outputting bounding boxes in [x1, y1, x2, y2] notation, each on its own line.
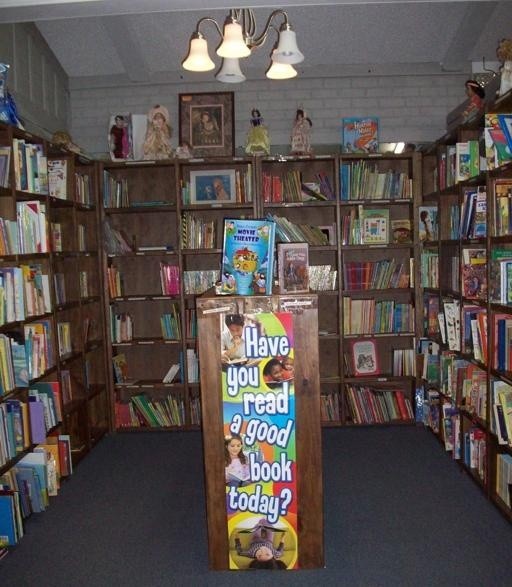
[496, 38, 511, 96]
[233, 518, 287, 569]
[262, 359, 294, 381]
[246, 108, 272, 157]
[193, 108, 223, 145]
[459, 79, 486, 119]
[140, 102, 172, 158]
[219, 313, 253, 372]
[107, 114, 131, 159]
[0, 62, 25, 130]
[287, 108, 316, 156]
[224, 431, 250, 485]
[281, 355, 294, 370]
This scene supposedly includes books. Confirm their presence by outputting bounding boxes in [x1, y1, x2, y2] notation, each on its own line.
[238, 523, 287, 533]
[340, 159, 413, 246]
[184, 270, 221, 295]
[340, 114, 381, 153]
[108, 264, 122, 298]
[263, 376, 294, 389]
[1, 135, 89, 255]
[308, 264, 337, 291]
[344, 258, 414, 290]
[103, 171, 130, 208]
[263, 169, 337, 246]
[221, 355, 249, 370]
[220, 218, 310, 296]
[316, 296, 419, 428]
[159, 262, 180, 296]
[181, 214, 216, 250]
[226, 469, 251, 487]
[1, 264, 92, 549]
[181, 163, 252, 205]
[107, 300, 203, 429]
[418, 106, 512, 509]
[104, 222, 134, 257]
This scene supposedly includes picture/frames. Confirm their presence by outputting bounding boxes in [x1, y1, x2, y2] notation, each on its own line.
[349, 339, 381, 378]
[177, 88, 236, 159]
[189, 168, 238, 205]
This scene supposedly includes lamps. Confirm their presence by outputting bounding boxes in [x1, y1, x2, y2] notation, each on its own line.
[175, 0, 306, 84]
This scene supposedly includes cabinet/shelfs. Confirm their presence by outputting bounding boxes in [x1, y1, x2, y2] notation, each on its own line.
[195, 281, 330, 573]
[176, 155, 257, 433]
[45, 138, 110, 461]
[423, 93, 511, 524]
[339, 147, 421, 426]
[0, 122, 72, 556]
[262, 152, 344, 427]
[98, 159, 191, 432]
